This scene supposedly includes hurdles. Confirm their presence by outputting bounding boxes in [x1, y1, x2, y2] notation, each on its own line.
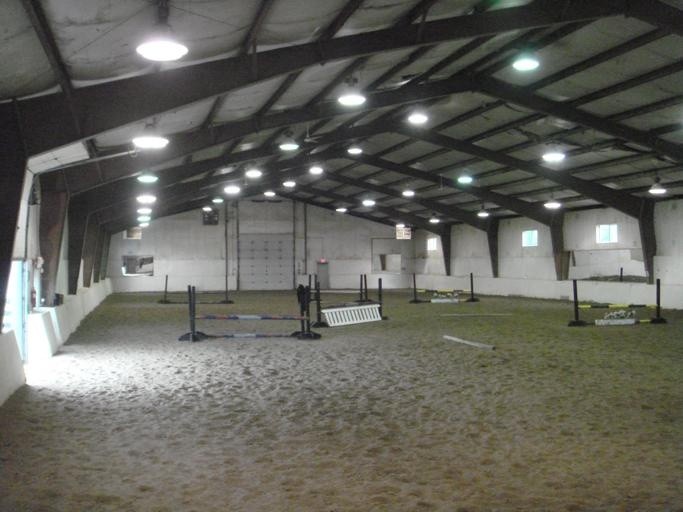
[179, 284, 320, 341]
[158, 273, 235, 304]
[567, 279, 667, 328]
[313, 279, 390, 327]
[307, 274, 376, 308]
[410, 272, 479, 303]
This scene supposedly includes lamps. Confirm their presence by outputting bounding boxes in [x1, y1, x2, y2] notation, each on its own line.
[648, 171, 666, 195]
[512, 49, 541, 73]
[278, 132, 299, 152]
[137, 171, 159, 184]
[134, 19, 189, 62]
[133, 125, 170, 151]
[409, 105, 429, 126]
[477, 204, 489, 219]
[429, 213, 440, 224]
[338, 72, 367, 106]
[347, 139, 363, 155]
[542, 140, 565, 164]
[543, 191, 561, 210]
